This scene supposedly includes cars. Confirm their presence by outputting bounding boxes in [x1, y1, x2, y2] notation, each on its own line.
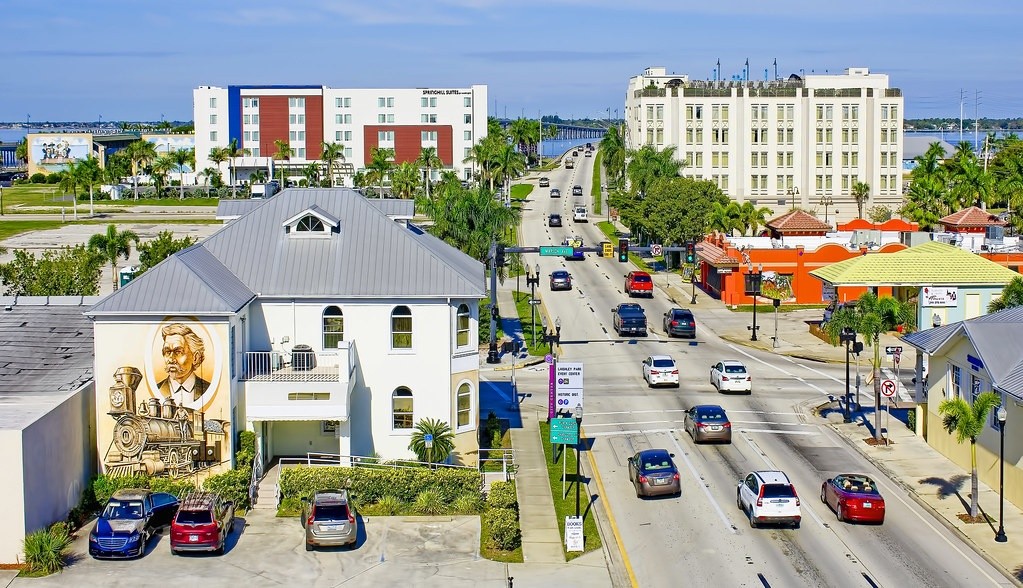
[549, 270, 572, 291]
[89, 488, 181, 561]
[820, 473, 885, 525]
[642, 355, 680, 388]
[710, 360, 752, 395]
[684, 404, 732, 445]
[627, 448, 682, 499]
[538, 143, 596, 227]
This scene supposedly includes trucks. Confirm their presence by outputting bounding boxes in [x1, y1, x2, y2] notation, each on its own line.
[572, 204, 588, 223]
[562, 236, 585, 261]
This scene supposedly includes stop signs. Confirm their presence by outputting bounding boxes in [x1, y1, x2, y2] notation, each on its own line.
[895, 349, 901, 364]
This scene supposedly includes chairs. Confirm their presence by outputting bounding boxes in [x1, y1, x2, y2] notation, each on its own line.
[646, 463, 652, 468]
[663, 461, 668, 466]
[702, 415, 722, 419]
[845, 481, 872, 492]
[738, 369, 743, 372]
[727, 369, 731, 372]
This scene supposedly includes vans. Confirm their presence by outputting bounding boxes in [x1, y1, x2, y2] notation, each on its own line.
[623, 271, 654, 298]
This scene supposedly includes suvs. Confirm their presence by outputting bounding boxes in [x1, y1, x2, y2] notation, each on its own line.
[300, 487, 358, 551]
[663, 307, 696, 338]
[736, 469, 802, 530]
[169, 489, 238, 555]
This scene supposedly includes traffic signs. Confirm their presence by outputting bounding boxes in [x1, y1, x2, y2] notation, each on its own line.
[886, 347, 903, 354]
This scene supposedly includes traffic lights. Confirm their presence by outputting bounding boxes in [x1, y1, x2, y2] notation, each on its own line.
[618, 239, 629, 264]
[686, 241, 695, 263]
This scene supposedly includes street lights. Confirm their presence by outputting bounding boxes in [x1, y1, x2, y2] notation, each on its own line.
[820, 195, 833, 223]
[749, 262, 761, 341]
[786, 185, 800, 209]
[525, 263, 541, 351]
[606, 106, 619, 123]
[997, 408, 1010, 542]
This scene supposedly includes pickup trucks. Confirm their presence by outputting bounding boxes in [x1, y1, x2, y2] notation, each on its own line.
[611, 303, 648, 336]
